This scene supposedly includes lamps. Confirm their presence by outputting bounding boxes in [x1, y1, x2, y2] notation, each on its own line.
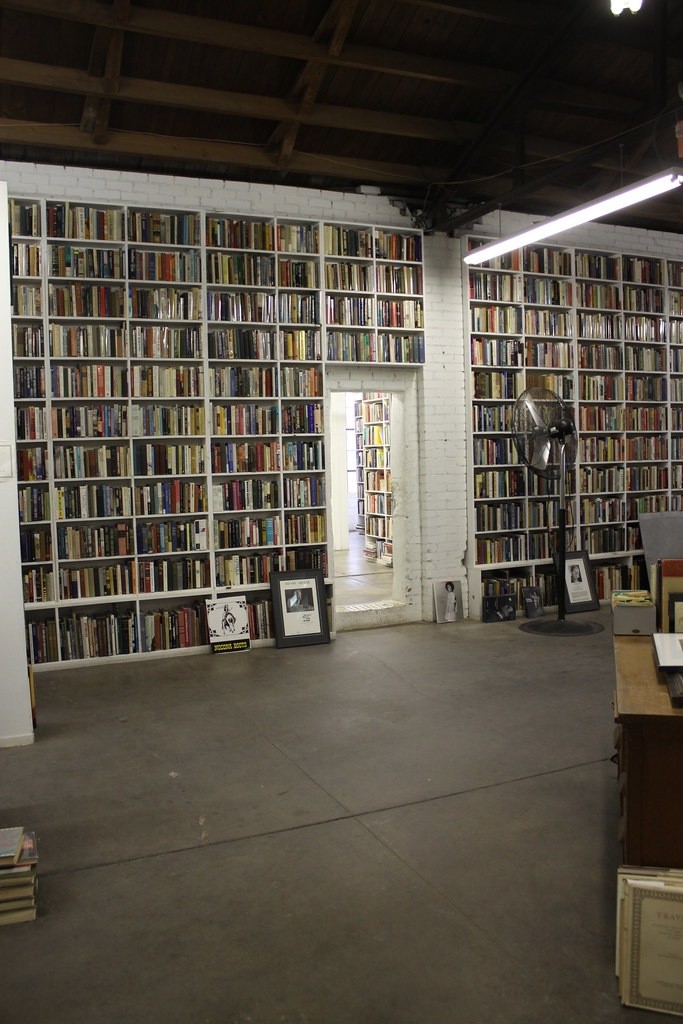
[462, 137, 683, 264]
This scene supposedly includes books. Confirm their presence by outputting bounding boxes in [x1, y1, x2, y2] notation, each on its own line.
[662, 672, 683, 709]
[6, 194, 683, 672]
[614, 863, 682, 1018]
[0, 826, 43, 928]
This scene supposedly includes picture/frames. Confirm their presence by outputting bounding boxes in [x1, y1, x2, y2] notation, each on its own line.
[432, 581, 463, 624]
[552, 550, 601, 615]
[270, 569, 330, 649]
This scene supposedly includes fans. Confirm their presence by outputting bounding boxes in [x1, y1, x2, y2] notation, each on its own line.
[510, 384, 606, 636]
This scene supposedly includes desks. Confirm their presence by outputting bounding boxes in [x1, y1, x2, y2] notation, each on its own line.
[612, 634, 683, 869]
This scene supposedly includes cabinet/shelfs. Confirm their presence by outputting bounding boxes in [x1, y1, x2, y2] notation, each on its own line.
[463, 233, 683, 619]
[9, 195, 417, 647]
[356, 390, 392, 568]
